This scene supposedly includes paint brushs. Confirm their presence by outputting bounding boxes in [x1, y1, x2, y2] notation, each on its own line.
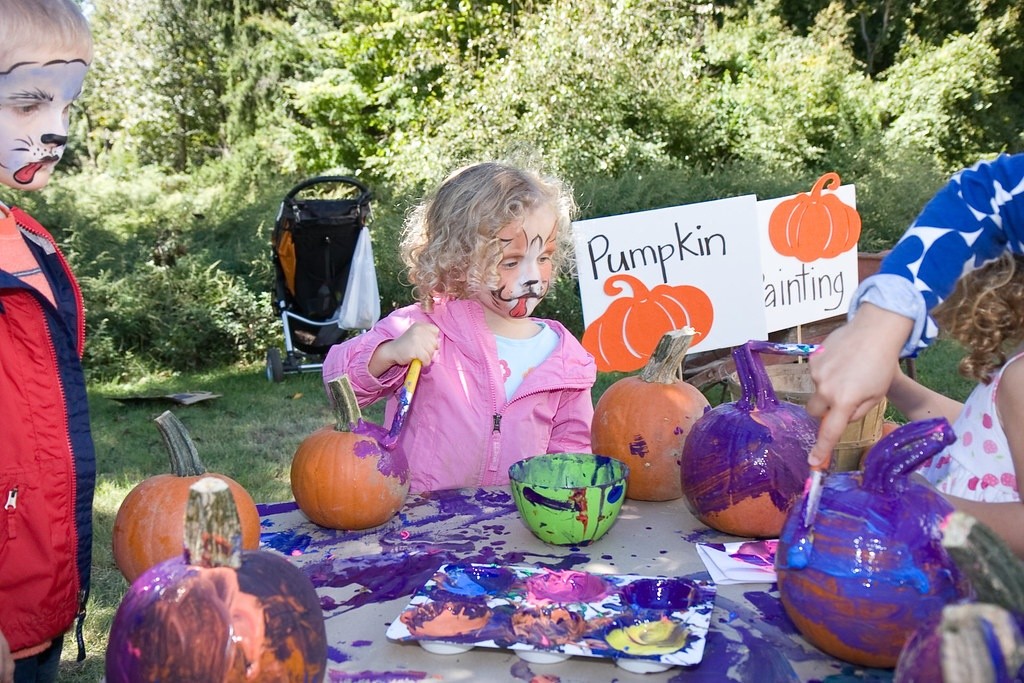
[381, 360, 421, 448]
[787, 406, 830, 569]
[748, 339, 821, 356]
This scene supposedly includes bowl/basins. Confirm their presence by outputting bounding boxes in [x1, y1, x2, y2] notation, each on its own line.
[507, 449, 631, 545]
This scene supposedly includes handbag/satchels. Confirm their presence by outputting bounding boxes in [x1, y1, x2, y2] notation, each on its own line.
[339, 222, 382, 332]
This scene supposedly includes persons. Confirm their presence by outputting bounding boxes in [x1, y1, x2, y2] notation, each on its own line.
[322, 163, 597, 492]
[805, 155, 1024, 555]
[0, 0, 98, 683]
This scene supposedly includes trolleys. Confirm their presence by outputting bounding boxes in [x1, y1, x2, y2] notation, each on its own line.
[675, 250, 918, 404]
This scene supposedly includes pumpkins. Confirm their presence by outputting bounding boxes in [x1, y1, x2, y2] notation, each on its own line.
[590, 325, 711, 503]
[776, 418, 1023, 683]
[291, 376, 410, 530]
[679, 342, 823, 535]
[107, 477, 327, 683]
[112, 411, 261, 582]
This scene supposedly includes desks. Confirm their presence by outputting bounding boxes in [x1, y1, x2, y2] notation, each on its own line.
[254, 486, 896, 683]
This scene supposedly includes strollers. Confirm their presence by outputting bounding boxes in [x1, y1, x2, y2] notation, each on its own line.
[264, 175, 377, 384]
